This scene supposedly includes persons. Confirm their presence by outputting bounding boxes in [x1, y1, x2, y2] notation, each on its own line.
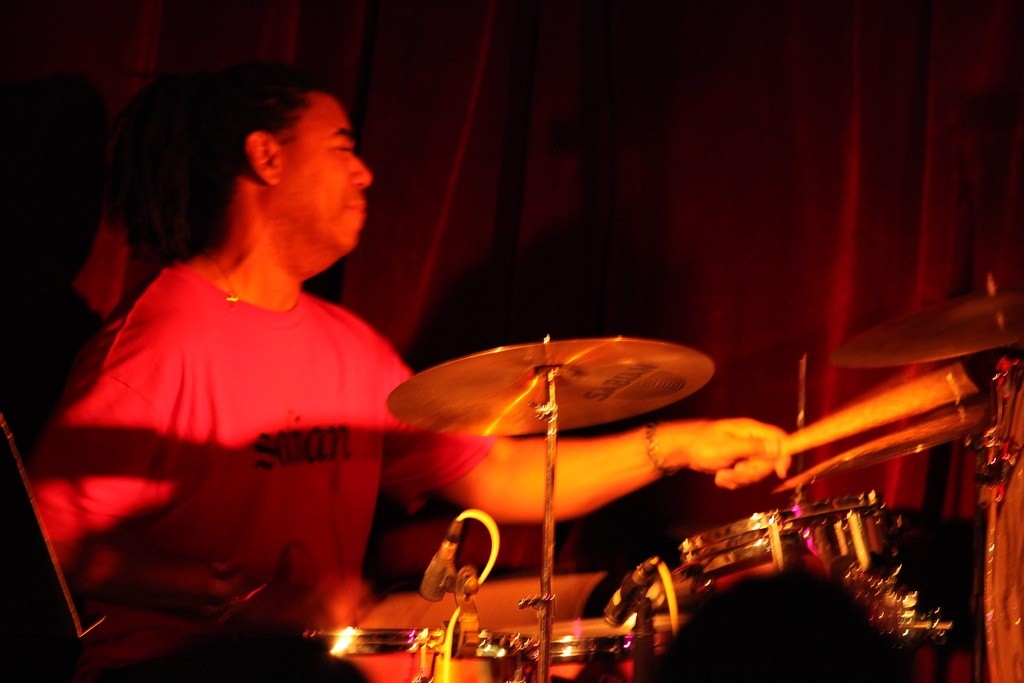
[27, 58, 792, 643]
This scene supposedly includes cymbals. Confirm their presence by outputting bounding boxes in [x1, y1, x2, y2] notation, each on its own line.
[829, 288, 1024, 375]
[383, 334, 717, 440]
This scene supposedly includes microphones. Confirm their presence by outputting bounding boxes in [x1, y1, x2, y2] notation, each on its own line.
[426, 503, 464, 601]
[604, 555, 661, 630]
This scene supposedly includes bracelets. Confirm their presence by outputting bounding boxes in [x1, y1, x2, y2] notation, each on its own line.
[644, 421, 677, 478]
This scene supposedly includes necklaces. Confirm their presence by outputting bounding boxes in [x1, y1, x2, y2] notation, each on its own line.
[202, 250, 237, 299]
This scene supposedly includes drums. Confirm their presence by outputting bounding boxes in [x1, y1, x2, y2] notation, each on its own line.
[785, 485, 901, 588]
[489, 607, 689, 682]
[297, 619, 551, 683]
[675, 487, 794, 596]
